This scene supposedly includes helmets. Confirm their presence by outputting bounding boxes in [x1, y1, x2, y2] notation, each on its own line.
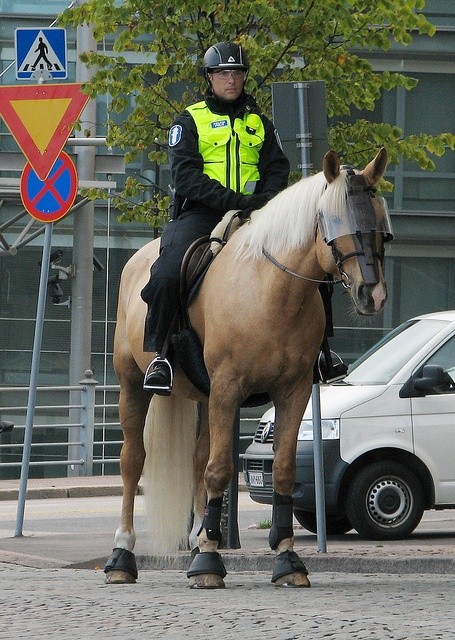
[201, 40, 249, 73]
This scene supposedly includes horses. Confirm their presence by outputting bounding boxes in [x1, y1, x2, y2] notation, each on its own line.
[103, 146, 395, 589]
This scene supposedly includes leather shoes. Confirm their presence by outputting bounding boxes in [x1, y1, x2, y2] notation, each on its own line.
[314, 352, 343, 384]
[143, 360, 172, 401]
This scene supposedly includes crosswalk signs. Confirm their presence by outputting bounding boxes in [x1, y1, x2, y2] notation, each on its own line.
[15, 27, 68, 80]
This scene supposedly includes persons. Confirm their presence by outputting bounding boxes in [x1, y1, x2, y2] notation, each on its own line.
[140, 40, 348, 396]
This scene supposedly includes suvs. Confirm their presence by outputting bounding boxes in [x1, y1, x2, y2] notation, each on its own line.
[238, 310, 454, 539]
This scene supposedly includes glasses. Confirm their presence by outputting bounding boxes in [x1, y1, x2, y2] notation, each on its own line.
[209, 71, 248, 81]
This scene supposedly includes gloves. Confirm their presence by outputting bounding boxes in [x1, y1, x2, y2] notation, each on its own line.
[233, 192, 266, 212]
